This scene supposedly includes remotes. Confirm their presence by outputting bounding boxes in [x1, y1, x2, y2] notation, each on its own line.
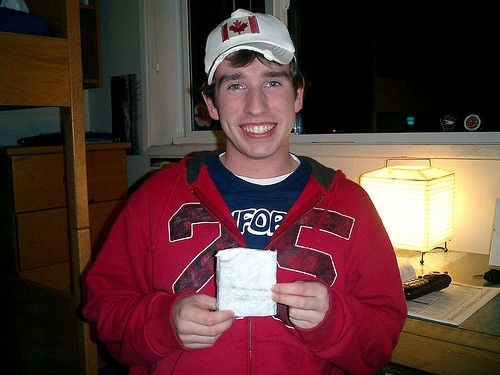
[400, 273, 452, 301]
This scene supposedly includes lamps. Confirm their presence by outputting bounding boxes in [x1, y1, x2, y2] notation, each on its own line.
[359, 164, 456, 265]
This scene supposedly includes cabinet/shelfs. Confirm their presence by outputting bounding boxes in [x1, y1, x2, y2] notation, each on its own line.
[0, 141, 131, 274]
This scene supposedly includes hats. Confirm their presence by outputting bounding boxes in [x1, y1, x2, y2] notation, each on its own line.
[204, 8, 295, 86]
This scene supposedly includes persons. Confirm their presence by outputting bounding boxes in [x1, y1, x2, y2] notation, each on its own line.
[81, 8, 408, 375]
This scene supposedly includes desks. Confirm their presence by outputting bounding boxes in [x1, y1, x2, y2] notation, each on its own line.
[19, 250, 500, 375]
